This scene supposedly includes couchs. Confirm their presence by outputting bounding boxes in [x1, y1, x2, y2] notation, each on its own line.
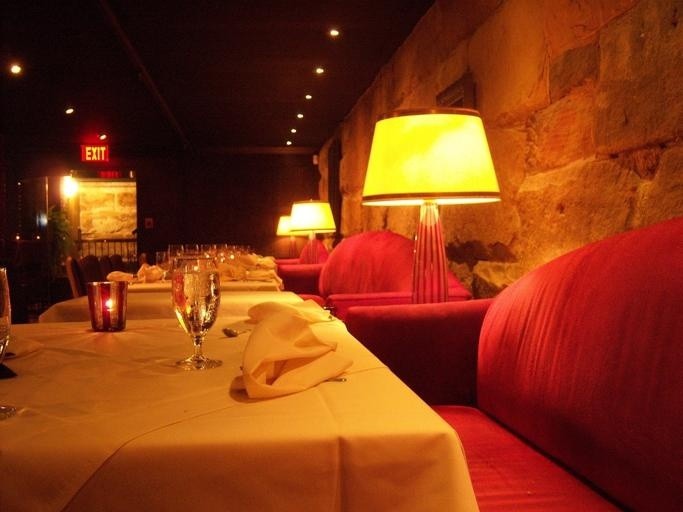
[344, 216, 683, 511]
[279, 230, 471, 317]
[273, 240, 328, 265]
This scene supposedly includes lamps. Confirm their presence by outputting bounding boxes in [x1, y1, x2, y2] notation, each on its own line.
[276, 216, 308, 243]
[289, 202, 337, 241]
[362, 107, 500, 304]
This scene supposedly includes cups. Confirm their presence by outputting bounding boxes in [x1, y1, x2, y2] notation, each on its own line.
[172, 268, 223, 369]
[0, 269, 15, 416]
[155, 244, 248, 281]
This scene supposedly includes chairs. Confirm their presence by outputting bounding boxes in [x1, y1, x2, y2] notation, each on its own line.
[66, 255, 124, 297]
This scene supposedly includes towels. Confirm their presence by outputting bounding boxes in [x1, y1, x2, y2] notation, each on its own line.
[136, 263, 165, 284]
[107, 269, 134, 285]
[248, 299, 333, 325]
[245, 269, 275, 282]
[241, 309, 354, 400]
